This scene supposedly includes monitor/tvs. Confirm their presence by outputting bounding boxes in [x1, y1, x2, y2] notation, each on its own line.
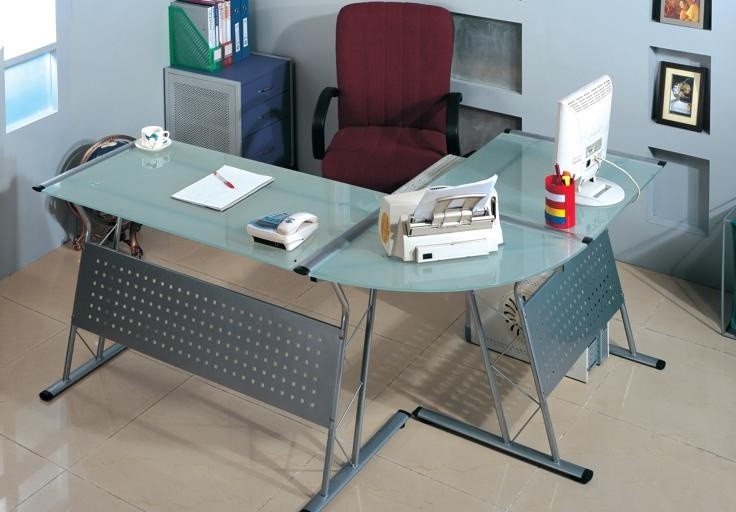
[553, 74, 625, 207]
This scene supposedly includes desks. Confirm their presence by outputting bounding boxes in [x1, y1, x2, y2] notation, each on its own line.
[30, 127, 665, 512]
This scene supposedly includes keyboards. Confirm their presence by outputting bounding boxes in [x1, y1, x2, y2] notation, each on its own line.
[390, 154, 467, 195]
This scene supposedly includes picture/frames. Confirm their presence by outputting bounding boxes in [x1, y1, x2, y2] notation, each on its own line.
[656, 60, 707, 133]
[651, 0, 712, 30]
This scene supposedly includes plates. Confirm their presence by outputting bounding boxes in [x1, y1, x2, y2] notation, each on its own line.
[133, 137, 172, 152]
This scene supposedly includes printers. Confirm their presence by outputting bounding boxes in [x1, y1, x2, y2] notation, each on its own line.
[378, 185, 504, 263]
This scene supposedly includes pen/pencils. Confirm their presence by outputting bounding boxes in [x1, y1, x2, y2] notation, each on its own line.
[554, 163, 575, 186]
[214, 172, 235, 188]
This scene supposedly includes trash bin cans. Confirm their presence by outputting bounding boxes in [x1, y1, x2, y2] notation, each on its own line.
[721, 206, 736, 340]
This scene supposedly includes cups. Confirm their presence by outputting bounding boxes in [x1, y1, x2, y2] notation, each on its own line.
[544, 175, 576, 229]
[140, 125, 170, 147]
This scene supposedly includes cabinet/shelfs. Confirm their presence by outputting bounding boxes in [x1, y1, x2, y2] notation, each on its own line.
[163, 50, 297, 168]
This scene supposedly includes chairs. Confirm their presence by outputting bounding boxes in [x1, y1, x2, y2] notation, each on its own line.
[310, 2, 461, 191]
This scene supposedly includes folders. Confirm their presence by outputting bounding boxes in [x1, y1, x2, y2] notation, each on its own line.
[231, 0, 249, 64]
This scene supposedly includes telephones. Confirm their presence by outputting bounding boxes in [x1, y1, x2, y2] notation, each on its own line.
[246, 212, 318, 251]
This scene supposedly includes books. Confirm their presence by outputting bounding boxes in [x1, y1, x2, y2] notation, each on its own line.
[171, 0, 252, 73]
[171, 164, 274, 213]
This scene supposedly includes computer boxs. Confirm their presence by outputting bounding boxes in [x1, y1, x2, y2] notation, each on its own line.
[470, 265, 610, 384]
